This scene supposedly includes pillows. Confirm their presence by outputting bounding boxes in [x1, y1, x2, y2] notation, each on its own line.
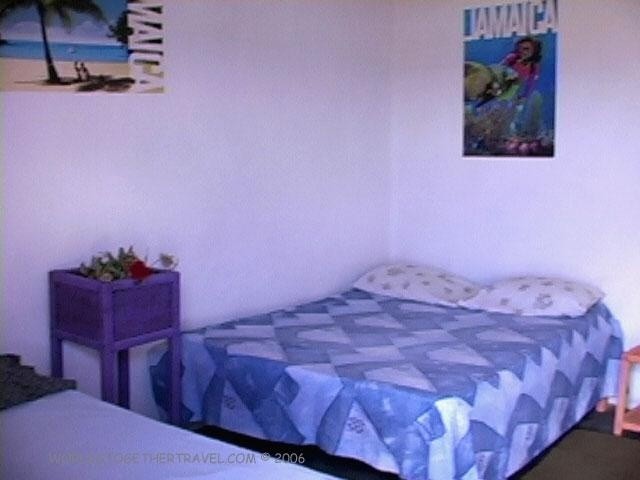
[182, 291, 624, 480]
[0, 353, 77, 412]
[457, 278, 604, 318]
[353, 264, 487, 308]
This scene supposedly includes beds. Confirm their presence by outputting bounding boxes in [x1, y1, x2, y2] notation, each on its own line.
[0, 388, 349, 480]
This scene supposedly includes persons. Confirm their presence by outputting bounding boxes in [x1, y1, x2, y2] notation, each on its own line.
[463, 35, 544, 139]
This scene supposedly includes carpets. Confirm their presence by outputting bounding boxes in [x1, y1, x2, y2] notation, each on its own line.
[520, 430, 640, 480]
[613, 346, 640, 437]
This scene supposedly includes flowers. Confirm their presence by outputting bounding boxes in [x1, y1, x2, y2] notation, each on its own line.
[70, 245, 178, 283]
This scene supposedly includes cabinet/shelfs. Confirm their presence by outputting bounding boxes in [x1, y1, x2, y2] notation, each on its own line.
[50, 265, 182, 427]
